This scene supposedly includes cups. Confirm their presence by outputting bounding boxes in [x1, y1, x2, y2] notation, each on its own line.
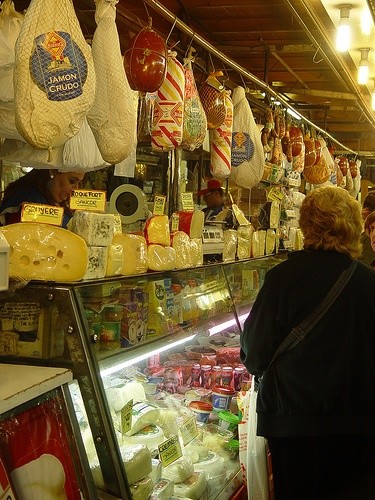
[225, 440, 239, 461]
[219, 411, 239, 432]
[189, 400, 213, 426]
[212, 385, 235, 409]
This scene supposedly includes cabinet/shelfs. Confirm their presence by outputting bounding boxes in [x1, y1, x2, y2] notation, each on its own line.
[0, 250, 295, 500]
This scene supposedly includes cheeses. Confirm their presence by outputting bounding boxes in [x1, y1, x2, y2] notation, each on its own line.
[65, 379, 229, 500]
[0, 209, 204, 281]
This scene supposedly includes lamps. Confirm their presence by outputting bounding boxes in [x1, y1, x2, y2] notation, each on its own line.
[334, 4, 375, 112]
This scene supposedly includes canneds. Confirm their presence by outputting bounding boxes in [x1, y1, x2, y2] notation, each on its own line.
[84, 305, 123, 351]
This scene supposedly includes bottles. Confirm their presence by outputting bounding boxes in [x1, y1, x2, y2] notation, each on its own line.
[190, 364, 201, 387]
[200, 365, 211, 389]
[233, 368, 244, 392]
[221, 367, 234, 388]
[210, 366, 221, 389]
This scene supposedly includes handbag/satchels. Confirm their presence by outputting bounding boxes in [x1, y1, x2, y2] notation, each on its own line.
[256, 362, 331, 437]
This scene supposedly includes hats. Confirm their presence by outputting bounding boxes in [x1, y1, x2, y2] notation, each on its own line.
[364, 211, 375, 236]
[195, 178, 221, 195]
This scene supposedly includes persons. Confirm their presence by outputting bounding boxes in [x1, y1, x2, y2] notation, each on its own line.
[363, 211, 375, 252]
[195, 179, 240, 231]
[358, 189, 375, 268]
[241, 185, 374, 499]
[0, 168, 85, 229]
